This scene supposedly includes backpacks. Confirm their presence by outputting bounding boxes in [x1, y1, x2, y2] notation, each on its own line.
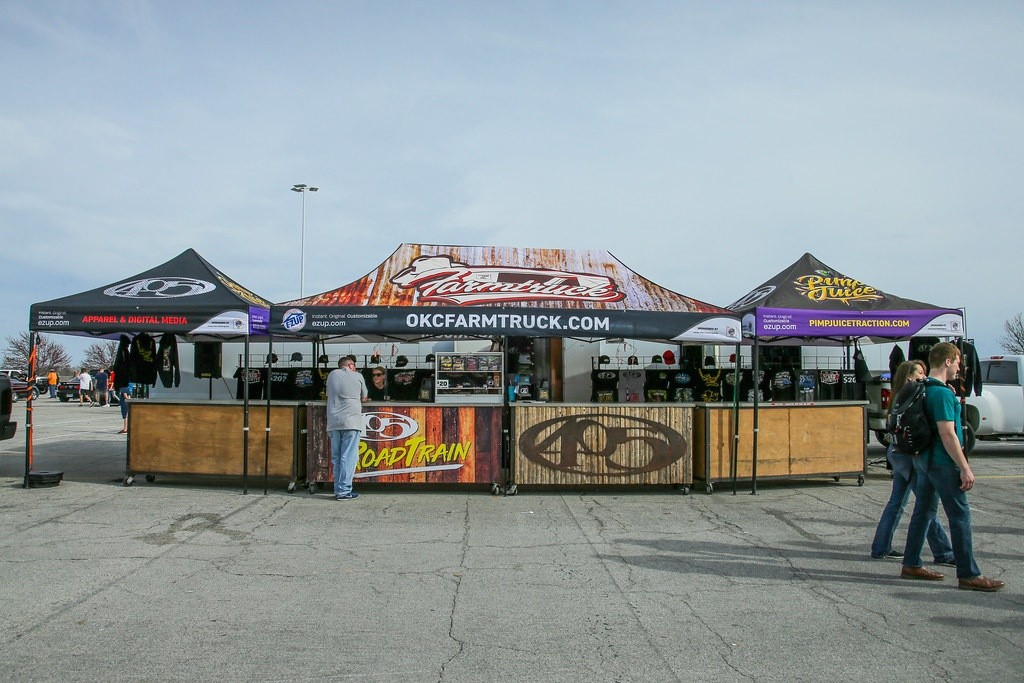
[887, 378, 950, 455]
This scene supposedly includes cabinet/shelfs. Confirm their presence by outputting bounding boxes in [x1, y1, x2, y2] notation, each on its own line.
[435, 352, 504, 403]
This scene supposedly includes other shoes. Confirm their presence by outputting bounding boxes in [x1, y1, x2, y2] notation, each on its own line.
[933, 553, 957, 568]
[89, 401, 94, 407]
[94, 403, 100, 407]
[871, 548, 905, 558]
[118, 430, 128, 434]
[102, 404, 110, 408]
[79, 404, 83, 406]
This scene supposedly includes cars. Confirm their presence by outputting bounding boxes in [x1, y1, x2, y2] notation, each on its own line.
[57, 369, 110, 402]
[9, 379, 39, 403]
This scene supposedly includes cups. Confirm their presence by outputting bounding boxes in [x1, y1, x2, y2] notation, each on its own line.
[507, 386, 516, 401]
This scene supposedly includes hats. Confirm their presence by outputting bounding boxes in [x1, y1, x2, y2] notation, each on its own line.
[705, 356, 715, 366]
[628, 356, 638, 365]
[651, 355, 662, 363]
[599, 355, 610, 364]
[425, 354, 435, 363]
[265, 354, 278, 364]
[290, 353, 302, 361]
[50, 368, 55, 371]
[663, 351, 675, 364]
[395, 356, 408, 367]
[730, 353, 736, 362]
[347, 355, 356, 363]
[369, 355, 381, 364]
[679, 355, 693, 366]
[318, 355, 329, 363]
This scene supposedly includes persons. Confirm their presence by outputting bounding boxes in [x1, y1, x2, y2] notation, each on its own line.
[901, 342, 1006, 592]
[870, 360, 956, 568]
[325, 357, 368, 501]
[18, 370, 27, 381]
[47, 369, 60, 398]
[367, 366, 386, 401]
[76, 366, 133, 434]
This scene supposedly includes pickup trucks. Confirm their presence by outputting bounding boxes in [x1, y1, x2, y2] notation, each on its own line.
[867, 355, 1024, 456]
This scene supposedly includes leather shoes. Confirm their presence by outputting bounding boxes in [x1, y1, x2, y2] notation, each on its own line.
[901, 565, 944, 580]
[958, 574, 1005, 590]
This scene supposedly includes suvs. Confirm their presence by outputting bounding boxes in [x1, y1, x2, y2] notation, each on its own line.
[0, 369, 48, 395]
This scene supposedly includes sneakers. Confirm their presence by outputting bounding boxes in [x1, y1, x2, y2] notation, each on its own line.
[337, 489, 359, 499]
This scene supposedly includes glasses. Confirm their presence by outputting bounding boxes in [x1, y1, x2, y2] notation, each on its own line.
[372, 373, 383, 377]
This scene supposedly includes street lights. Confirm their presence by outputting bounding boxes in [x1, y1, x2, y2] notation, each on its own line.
[291, 184, 318, 298]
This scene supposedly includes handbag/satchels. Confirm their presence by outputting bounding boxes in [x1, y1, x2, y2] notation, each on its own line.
[56, 374, 59, 385]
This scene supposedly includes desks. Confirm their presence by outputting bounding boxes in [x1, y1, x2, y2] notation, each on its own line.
[124, 399, 869, 495]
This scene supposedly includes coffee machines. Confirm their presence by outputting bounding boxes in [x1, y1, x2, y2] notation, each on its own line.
[420, 377, 433, 402]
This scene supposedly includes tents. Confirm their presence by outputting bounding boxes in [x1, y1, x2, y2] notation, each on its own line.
[263, 243, 743, 495]
[724, 253, 968, 494]
[22, 249, 276, 495]
